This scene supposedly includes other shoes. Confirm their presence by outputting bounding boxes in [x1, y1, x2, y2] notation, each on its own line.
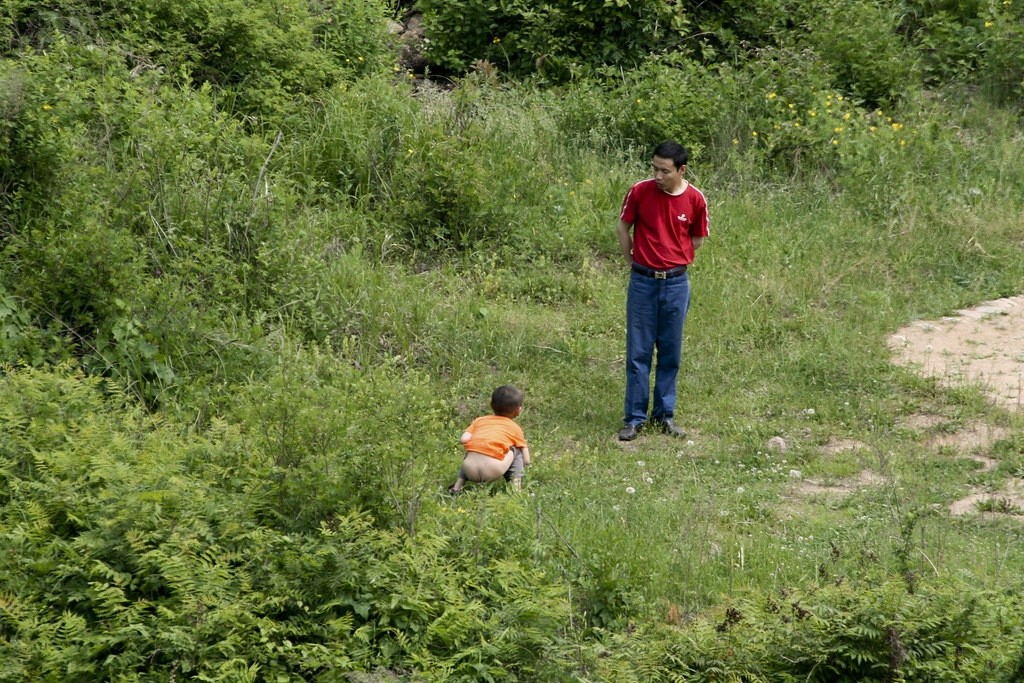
[448, 485, 462, 495]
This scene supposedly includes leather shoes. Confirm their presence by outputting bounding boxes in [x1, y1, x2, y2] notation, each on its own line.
[620, 423, 644, 440]
[650, 416, 686, 438]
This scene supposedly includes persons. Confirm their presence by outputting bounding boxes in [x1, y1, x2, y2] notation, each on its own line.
[447, 384, 531, 496]
[617, 142, 710, 442]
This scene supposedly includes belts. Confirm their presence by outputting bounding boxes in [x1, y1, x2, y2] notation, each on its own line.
[632, 264, 687, 279]
